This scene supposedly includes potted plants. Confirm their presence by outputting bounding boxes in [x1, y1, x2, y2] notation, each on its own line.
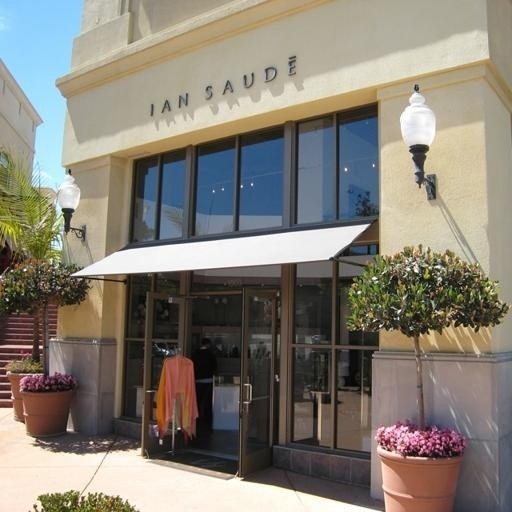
[343, 241, 509, 512]
[0, 259, 89, 437]
[0, 141, 71, 424]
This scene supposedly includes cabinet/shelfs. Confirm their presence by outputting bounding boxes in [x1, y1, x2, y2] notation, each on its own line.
[210, 371, 250, 432]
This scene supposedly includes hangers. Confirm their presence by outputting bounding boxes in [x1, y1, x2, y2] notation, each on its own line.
[164, 345, 192, 366]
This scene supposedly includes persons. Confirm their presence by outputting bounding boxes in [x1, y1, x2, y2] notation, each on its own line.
[192, 337, 223, 437]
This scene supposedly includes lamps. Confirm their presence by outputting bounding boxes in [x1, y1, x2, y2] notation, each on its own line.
[57, 167, 87, 244]
[400, 82, 440, 201]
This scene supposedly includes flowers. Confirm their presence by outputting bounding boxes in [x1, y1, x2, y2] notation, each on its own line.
[18, 373, 77, 394]
[374, 417, 470, 460]
